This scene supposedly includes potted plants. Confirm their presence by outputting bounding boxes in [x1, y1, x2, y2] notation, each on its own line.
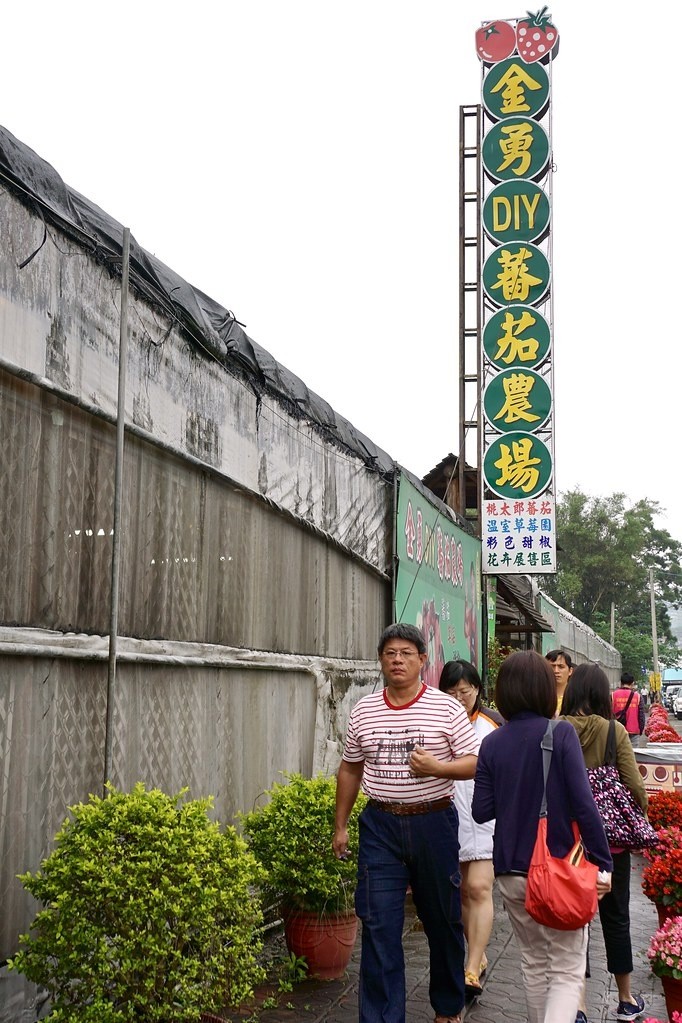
[232, 769, 369, 980]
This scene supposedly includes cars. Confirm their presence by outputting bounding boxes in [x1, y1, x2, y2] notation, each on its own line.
[664, 684, 682, 721]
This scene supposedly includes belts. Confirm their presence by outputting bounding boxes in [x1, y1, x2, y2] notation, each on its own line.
[370, 798, 455, 814]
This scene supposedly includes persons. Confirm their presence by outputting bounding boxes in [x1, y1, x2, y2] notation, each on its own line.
[610, 673, 645, 748]
[557, 663, 650, 1023]
[331, 623, 482, 1023]
[634, 686, 659, 705]
[471, 649, 613, 1023]
[464, 561, 478, 670]
[439, 659, 503, 995]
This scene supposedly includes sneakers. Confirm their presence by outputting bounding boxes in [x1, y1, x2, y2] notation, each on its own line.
[617, 995, 645, 1021]
[575, 1010, 587, 1023]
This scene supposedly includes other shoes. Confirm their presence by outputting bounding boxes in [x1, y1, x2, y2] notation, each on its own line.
[434, 1012, 461, 1023]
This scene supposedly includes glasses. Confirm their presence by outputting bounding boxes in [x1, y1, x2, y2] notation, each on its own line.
[451, 688, 475, 698]
[380, 650, 418, 657]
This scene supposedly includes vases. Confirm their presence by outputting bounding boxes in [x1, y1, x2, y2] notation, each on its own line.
[656, 905, 682, 929]
[660, 975, 682, 1023]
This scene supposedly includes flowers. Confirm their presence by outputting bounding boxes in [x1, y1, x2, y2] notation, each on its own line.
[642, 826, 682, 865]
[641, 844, 682, 914]
[644, 703, 682, 743]
[634, 914, 682, 983]
[647, 789, 682, 831]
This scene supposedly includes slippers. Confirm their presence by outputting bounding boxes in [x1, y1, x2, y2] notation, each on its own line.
[479, 956, 488, 976]
[464, 971, 482, 995]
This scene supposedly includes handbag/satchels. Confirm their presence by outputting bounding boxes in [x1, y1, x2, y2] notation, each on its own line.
[525, 812, 599, 930]
[614, 712, 626, 726]
[586, 720, 660, 847]
